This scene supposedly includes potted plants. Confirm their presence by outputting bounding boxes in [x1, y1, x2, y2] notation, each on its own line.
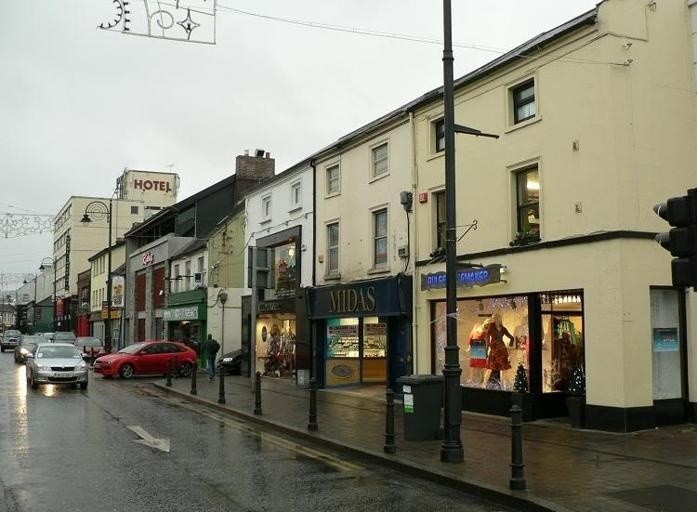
[565, 367, 586, 428]
[510, 363, 532, 422]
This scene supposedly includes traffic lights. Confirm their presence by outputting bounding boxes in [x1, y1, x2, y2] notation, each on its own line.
[666, 187, 697, 291]
[653, 202, 670, 252]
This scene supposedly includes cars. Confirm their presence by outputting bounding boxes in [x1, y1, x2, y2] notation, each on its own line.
[219, 347, 248, 375]
[93, 340, 198, 379]
[0, 329, 106, 390]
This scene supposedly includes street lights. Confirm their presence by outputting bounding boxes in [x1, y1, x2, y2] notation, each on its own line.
[22, 274, 36, 332]
[79, 200, 114, 356]
[38, 256, 57, 333]
[6, 290, 19, 330]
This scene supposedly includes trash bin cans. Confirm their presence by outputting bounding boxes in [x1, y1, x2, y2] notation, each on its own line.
[565, 396, 585, 428]
[396, 374, 446, 441]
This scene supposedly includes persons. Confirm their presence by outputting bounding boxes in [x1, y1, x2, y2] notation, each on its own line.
[465, 317, 490, 387]
[481, 313, 514, 388]
[201, 333, 220, 381]
